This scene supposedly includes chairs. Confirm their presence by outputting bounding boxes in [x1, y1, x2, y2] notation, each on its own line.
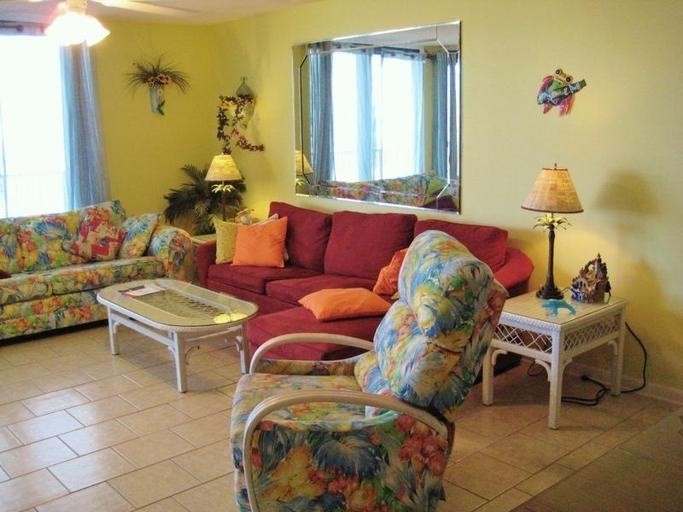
[228, 226, 510, 512]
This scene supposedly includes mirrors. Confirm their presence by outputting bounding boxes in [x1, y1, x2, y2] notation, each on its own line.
[289, 18, 461, 218]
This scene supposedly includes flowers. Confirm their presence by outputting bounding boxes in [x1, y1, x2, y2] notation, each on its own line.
[214, 93, 265, 154]
[128, 54, 190, 100]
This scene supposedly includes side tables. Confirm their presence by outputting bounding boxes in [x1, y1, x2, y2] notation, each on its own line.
[481, 290, 629, 431]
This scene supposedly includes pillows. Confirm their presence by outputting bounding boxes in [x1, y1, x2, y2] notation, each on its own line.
[69, 207, 164, 262]
[298, 246, 411, 322]
[211, 214, 290, 268]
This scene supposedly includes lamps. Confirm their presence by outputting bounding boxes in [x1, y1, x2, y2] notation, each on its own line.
[521, 162, 585, 301]
[295, 151, 314, 176]
[204, 154, 242, 222]
[39, 0, 111, 47]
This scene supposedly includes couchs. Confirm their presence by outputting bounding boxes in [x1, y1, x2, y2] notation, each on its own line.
[195, 201, 537, 385]
[316, 173, 459, 206]
[0, 200, 192, 342]
